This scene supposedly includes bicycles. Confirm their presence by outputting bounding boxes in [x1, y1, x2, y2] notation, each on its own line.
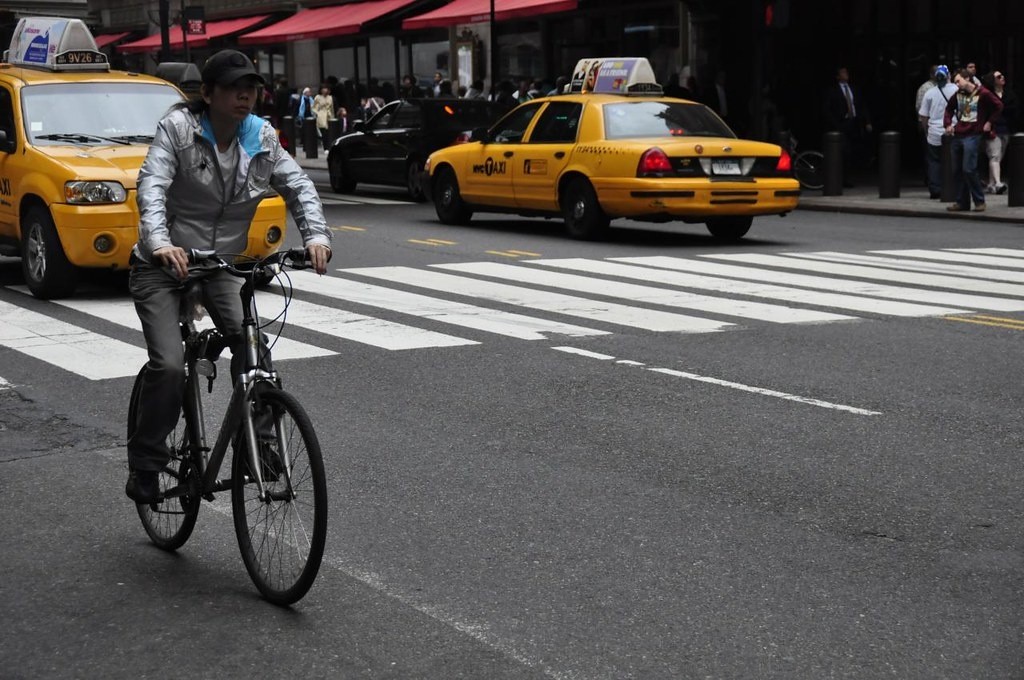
[126, 247, 334, 606]
[764, 125, 825, 191]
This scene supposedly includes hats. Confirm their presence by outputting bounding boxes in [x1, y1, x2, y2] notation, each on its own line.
[470, 81, 484, 91]
[500, 80, 513, 92]
[202, 50, 266, 87]
[935, 65, 948, 77]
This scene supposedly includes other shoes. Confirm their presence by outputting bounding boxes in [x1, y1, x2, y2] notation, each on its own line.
[127, 466, 160, 500]
[973, 202, 985, 211]
[988, 186, 995, 195]
[230, 438, 282, 478]
[996, 184, 1008, 194]
[324, 150, 329, 154]
[947, 203, 970, 211]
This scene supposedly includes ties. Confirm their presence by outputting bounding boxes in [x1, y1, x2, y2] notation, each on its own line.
[844, 86, 853, 120]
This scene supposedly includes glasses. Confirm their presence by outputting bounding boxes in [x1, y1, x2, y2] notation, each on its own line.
[994, 73, 1003, 80]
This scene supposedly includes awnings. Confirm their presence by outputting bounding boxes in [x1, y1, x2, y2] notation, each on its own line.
[237, 0, 416, 46]
[116, 14, 272, 54]
[95, 31, 131, 49]
[402, 0, 578, 30]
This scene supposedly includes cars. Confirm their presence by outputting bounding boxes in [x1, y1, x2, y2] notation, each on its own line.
[424, 57, 802, 243]
[327, 95, 526, 205]
[0, 15, 287, 301]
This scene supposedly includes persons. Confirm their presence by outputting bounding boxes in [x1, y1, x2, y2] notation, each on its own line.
[663, 53, 1017, 212]
[257, 71, 568, 150]
[125, 48, 333, 506]
